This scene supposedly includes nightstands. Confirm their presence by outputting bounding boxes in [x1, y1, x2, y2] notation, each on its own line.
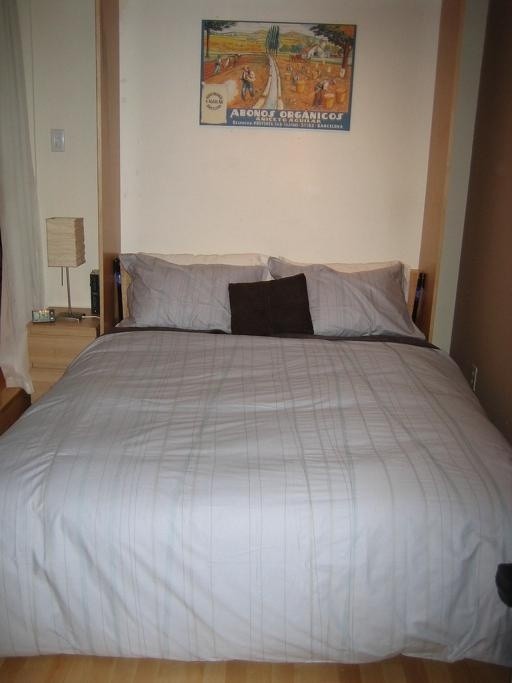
[23, 306, 99, 404]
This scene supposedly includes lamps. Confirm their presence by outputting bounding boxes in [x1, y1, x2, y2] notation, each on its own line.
[46, 216, 88, 320]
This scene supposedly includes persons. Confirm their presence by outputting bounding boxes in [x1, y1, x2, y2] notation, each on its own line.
[214, 54, 222, 74]
[311, 78, 337, 107]
[239, 65, 256, 103]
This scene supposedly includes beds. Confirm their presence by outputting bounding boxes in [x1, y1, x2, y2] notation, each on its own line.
[2, 251, 510, 662]
